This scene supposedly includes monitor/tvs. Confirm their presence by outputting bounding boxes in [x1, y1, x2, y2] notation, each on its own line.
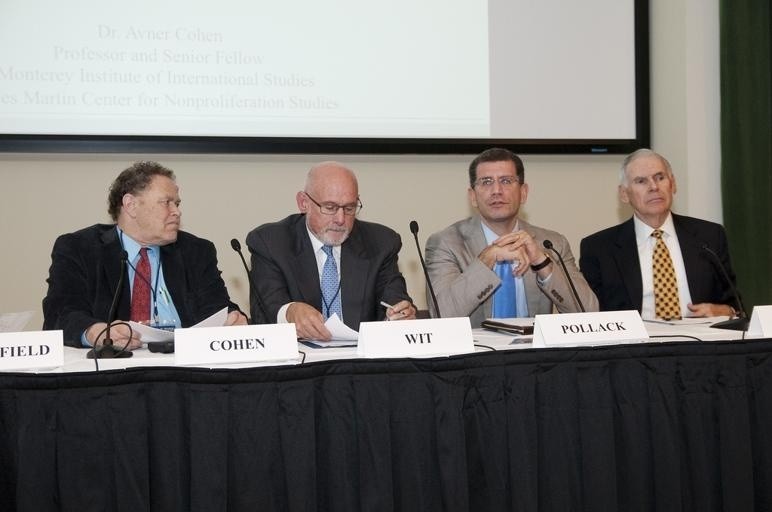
[481, 320, 534, 335]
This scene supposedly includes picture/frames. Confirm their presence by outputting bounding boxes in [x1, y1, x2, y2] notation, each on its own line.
[0, 308, 770, 512]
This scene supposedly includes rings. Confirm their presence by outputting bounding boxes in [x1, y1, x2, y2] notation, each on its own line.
[380, 301, 393, 308]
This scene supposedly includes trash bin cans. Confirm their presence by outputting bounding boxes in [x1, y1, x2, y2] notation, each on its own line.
[531, 254, 550, 271]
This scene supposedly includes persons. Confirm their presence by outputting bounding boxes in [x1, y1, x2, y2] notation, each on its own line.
[245, 161, 418, 342]
[42, 161, 249, 351]
[425, 147, 600, 329]
[579, 148, 745, 317]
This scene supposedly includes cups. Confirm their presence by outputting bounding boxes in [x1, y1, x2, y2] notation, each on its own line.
[474, 176, 519, 187]
[305, 193, 363, 216]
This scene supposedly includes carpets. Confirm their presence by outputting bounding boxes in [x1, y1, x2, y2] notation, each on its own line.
[651, 229, 681, 321]
[492, 258, 517, 319]
[130, 247, 151, 326]
[321, 244, 342, 322]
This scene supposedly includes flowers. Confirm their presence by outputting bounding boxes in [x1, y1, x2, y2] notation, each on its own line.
[87, 250, 132, 358]
[231, 239, 273, 324]
[543, 240, 585, 312]
[696, 240, 749, 331]
[410, 221, 441, 318]
